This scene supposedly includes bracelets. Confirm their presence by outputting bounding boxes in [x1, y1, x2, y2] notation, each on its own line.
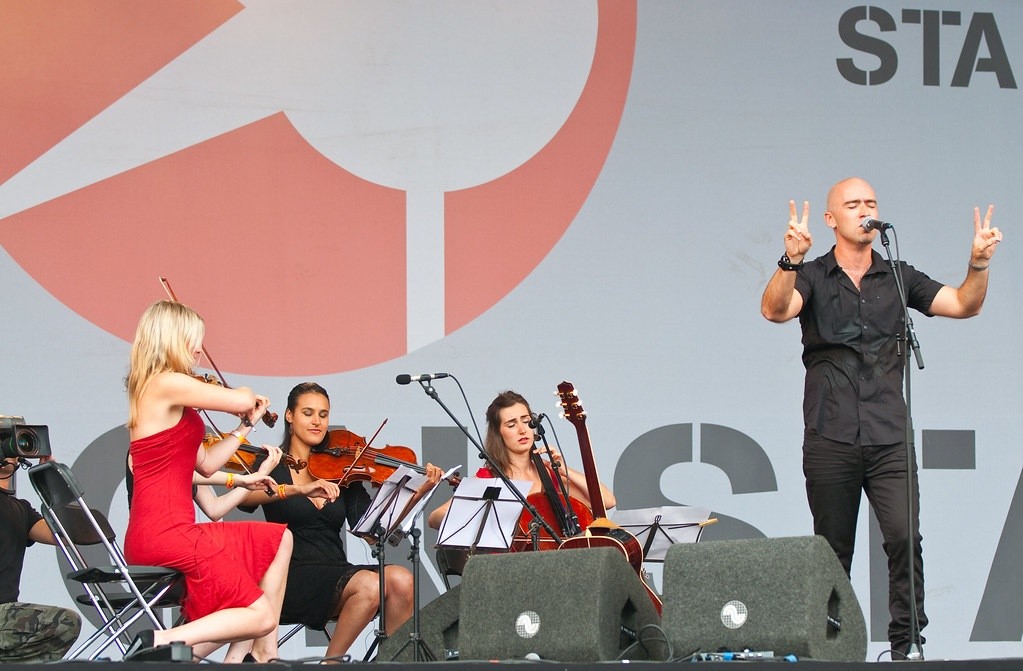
[226, 472, 234, 488]
[969, 262, 988, 271]
[278, 483, 288, 500]
[230, 430, 244, 445]
[778, 251, 804, 271]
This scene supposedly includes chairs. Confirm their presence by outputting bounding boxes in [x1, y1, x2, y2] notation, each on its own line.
[29, 461, 193, 660]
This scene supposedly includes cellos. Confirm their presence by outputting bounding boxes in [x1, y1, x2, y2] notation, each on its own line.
[516, 441, 595, 550]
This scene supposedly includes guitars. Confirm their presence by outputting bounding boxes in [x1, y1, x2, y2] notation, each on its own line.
[558, 381, 663, 613]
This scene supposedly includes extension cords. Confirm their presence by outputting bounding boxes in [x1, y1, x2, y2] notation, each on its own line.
[126, 645, 194, 662]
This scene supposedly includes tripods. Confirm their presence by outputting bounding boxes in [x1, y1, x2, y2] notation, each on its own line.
[389, 524, 439, 663]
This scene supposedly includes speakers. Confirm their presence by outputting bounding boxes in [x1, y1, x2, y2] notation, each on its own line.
[457, 545, 663, 663]
[379, 584, 460, 663]
[658, 533, 868, 662]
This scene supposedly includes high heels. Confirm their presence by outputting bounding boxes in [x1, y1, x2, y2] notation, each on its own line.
[243, 653, 256, 663]
[122, 628, 154, 660]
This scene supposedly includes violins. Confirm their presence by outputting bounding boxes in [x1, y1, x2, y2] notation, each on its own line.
[190, 371, 277, 427]
[206, 432, 307, 473]
[307, 430, 460, 486]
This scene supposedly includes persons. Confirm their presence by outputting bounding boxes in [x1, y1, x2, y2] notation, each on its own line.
[125, 445, 254, 664]
[233, 381, 446, 664]
[428, 388, 616, 550]
[0, 451, 82, 660]
[121, 298, 293, 662]
[761, 176, 1003, 661]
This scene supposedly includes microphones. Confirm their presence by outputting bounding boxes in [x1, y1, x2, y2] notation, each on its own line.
[396, 373, 450, 385]
[862, 217, 893, 231]
[528, 413, 545, 429]
